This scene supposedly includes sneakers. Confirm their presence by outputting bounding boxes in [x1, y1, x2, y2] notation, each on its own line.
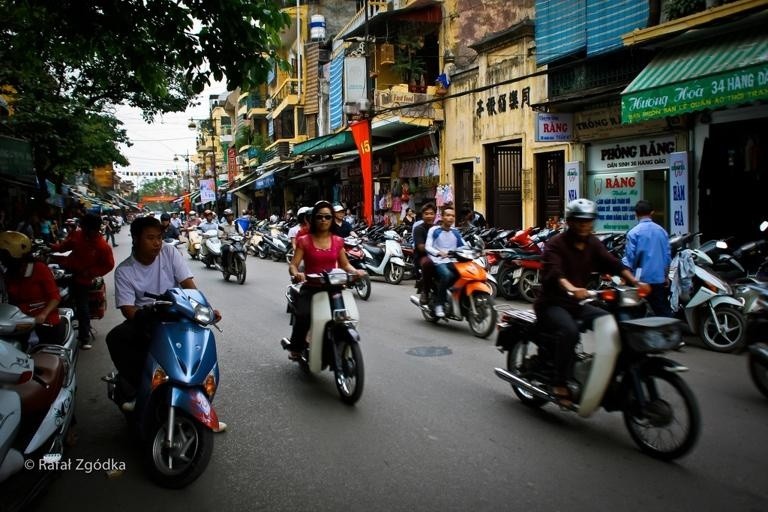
[123, 400, 135, 411]
[288, 351, 303, 361]
[83, 335, 92, 350]
[435, 305, 445, 318]
[419, 291, 429, 305]
[213, 421, 226, 434]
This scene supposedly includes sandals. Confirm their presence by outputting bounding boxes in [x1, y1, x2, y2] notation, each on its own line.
[552, 387, 574, 412]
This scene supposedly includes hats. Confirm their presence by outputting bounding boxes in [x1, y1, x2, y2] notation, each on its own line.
[333, 205, 347, 212]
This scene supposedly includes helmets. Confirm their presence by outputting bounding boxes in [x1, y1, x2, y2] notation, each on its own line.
[563, 197, 598, 223]
[0, 230, 32, 258]
[224, 209, 234, 216]
[161, 213, 170, 221]
[203, 210, 213, 218]
[296, 206, 314, 222]
[189, 210, 196, 215]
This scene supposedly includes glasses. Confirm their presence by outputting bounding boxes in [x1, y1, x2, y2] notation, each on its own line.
[314, 214, 333, 220]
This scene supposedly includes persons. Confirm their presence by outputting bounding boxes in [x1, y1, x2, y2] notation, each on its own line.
[402, 207, 416, 226]
[622, 198, 686, 350]
[2, 200, 370, 434]
[413, 202, 438, 305]
[533, 197, 651, 408]
[463, 208, 486, 229]
[424, 206, 470, 318]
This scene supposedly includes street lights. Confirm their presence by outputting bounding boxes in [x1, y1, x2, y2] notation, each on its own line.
[189, 116, 220, 221]
[174, 150, 193, 194]
